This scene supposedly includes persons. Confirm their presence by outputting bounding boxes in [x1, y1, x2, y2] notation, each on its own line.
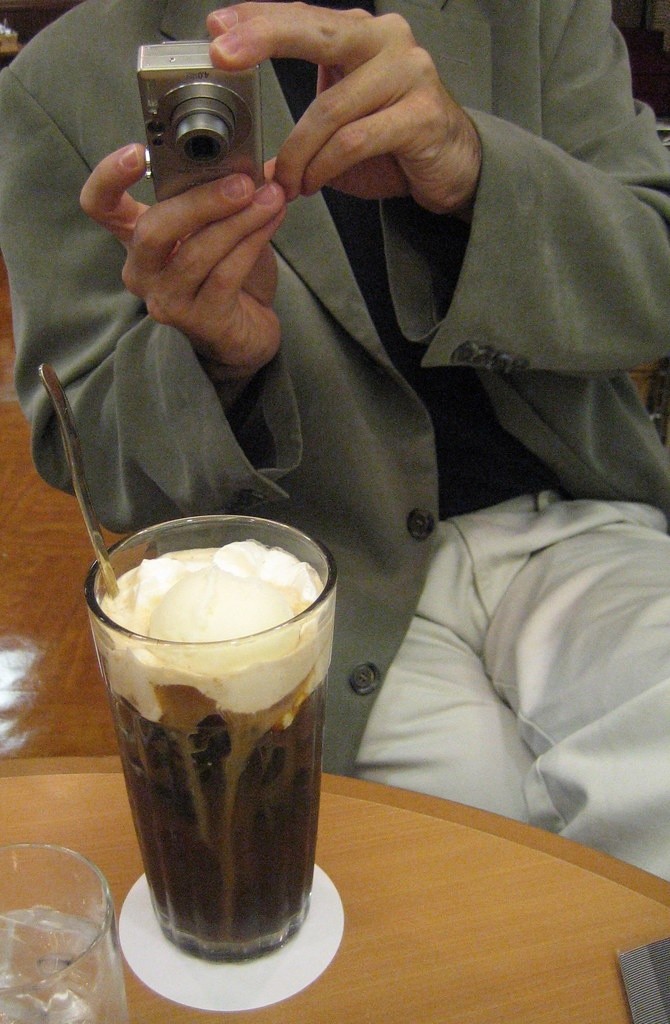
[0, 0, 670, 881]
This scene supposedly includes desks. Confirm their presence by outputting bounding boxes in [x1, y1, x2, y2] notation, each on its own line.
[0, 654, 632, 1024]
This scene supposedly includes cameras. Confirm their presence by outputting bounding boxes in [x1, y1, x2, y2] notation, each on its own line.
[135, 39, 264, 195]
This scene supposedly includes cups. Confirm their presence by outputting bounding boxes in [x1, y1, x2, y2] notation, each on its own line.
[1, 842, 128, 1023]
[84, 510, 336, 958]
